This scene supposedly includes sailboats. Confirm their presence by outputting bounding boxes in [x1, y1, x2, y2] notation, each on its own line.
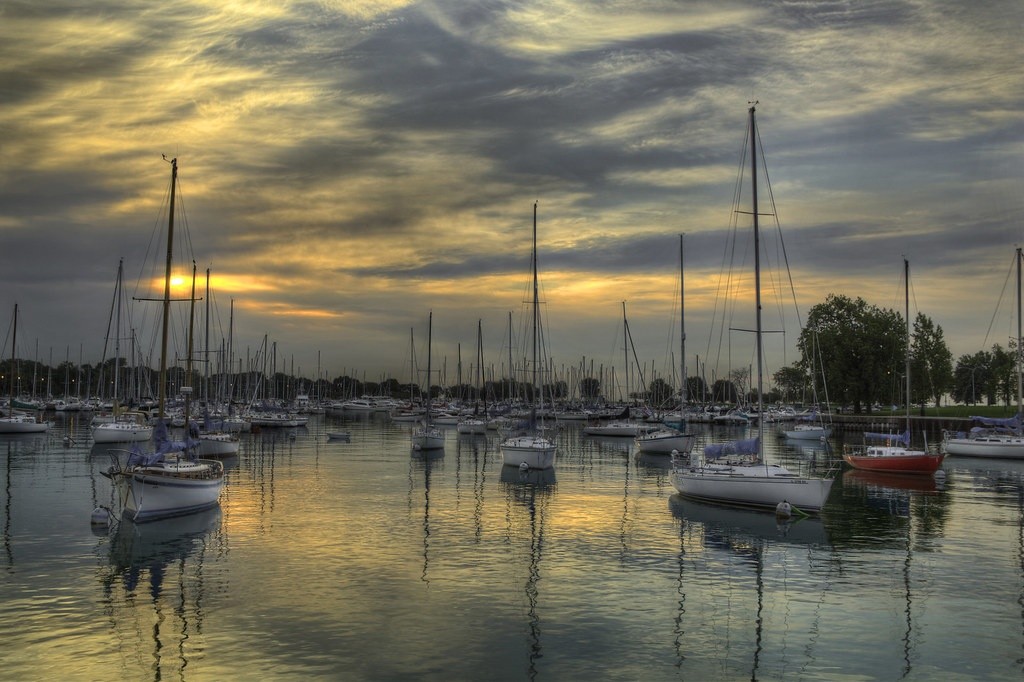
[664, 98, 844, 514]
[95, 153, 225, 523]
[0, 98, 1024, 458]
[841, 257, 948, 477]
